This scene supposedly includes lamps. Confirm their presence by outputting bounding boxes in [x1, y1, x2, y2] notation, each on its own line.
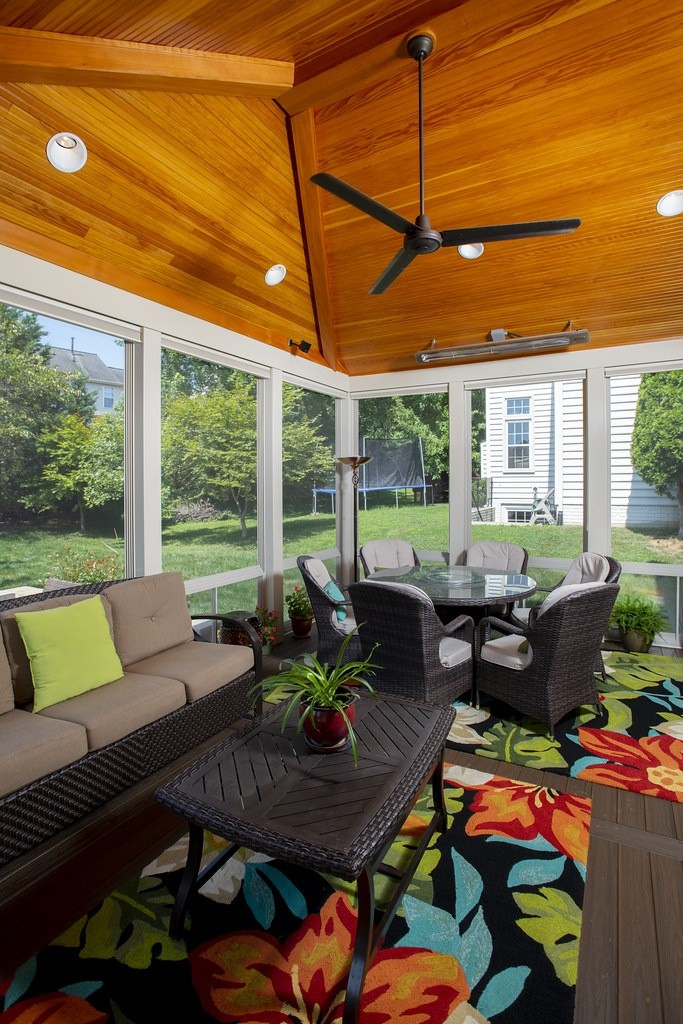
[416, 320, 590, 364]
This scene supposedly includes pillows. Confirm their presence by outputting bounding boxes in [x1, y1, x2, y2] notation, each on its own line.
[13, 594, 124, 714]
[323, 581, 347, 622]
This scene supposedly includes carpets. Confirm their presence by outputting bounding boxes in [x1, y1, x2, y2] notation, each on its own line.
[0, 761, 593, 1024]
[446, 650, 683, 804]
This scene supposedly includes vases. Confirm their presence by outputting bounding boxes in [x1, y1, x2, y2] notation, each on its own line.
[262, 637, 274, 656]
[288, 613, 314, 639]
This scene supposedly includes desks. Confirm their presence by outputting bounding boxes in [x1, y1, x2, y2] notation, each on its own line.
[152, 686, 457, 1024]
[366, 565, 538, 658]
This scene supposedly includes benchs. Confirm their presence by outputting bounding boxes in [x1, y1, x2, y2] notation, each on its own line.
[0, 572, 263, 910]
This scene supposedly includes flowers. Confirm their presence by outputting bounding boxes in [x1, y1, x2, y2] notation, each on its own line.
[252, 604, 284, 647]
[284, 585, 314, 619]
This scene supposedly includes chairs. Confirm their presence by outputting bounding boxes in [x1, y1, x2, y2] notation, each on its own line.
[464, 540, 528, 621]
[475, 581, 620, 742]
[296, 555, 362, 679]
[347, 578, 475, 707]
[508, 551, 623, 686]
[359, 539, 422, 578]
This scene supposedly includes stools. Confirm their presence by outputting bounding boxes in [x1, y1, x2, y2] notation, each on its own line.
[217, 610, 263, 648]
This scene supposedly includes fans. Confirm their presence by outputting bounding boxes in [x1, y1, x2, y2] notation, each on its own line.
[309, 35, 581, 294]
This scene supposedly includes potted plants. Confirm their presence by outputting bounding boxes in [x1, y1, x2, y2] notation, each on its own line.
[607, 590, 672, 653]
[245, 619, 386, 770]
